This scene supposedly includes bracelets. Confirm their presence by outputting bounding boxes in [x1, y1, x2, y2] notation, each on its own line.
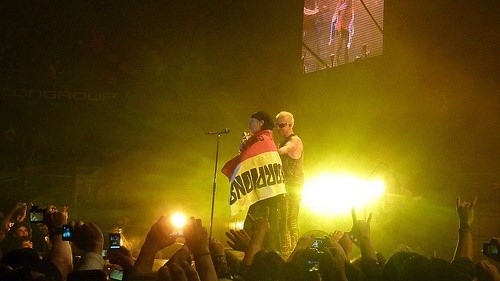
[459, 227, 472, 233]
[193, 253, 210, 260]
[50, 227, 62, 237]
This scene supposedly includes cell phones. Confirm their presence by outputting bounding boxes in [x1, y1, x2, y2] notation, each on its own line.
[108, 233, 120, 263]
[311, 237, 325, 248]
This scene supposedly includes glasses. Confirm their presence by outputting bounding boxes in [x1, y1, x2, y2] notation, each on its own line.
[276, 122, 292, 128]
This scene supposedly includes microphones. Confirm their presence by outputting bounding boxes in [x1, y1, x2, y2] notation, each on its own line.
[207, 128, 230, 135]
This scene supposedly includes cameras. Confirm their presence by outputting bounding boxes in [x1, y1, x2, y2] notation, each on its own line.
[482, 243, 500, 257]
[29, 208, 47, 223]
[62, 225, 72, 241]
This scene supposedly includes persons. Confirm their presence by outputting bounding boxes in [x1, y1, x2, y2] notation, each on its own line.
[240, 111, 286, 251]
[303, 0, 327, 72]
[63, 228, 70, 237]
[0, 197, 500, 281]
[276, 111, 304, 251]
[330, 0, 354, 68]
